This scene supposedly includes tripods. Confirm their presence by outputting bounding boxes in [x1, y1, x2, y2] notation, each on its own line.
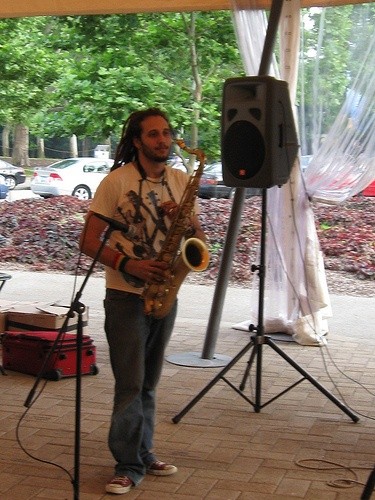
[172, 187, 360, 424]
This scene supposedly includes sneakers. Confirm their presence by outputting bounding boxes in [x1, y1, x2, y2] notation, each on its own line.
[145, 459, 178, 476]
[104, 471, 133, 494]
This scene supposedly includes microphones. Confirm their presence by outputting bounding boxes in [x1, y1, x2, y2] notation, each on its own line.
[92, 211, 141, 238]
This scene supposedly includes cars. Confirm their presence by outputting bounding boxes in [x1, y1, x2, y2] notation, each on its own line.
[31, 157, 125, 202]
[0, 174, 9, 199]
[0, 159, 26, 190]
[167, 157, 264, 199]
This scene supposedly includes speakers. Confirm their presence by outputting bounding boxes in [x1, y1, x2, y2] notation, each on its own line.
[222, 74, 299, 188]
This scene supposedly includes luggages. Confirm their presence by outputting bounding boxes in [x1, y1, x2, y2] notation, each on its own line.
[3, 328, 100, 382]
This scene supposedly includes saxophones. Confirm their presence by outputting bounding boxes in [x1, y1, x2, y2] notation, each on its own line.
[141, 138, 211, 319]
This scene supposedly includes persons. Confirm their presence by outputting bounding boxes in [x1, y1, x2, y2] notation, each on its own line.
[80, 109, 206, 494]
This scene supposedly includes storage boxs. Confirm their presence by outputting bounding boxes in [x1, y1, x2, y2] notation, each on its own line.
[0, 306, 89, 336]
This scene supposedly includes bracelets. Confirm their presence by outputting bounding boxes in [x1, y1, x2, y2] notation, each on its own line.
[113, 252, 130, 272]
